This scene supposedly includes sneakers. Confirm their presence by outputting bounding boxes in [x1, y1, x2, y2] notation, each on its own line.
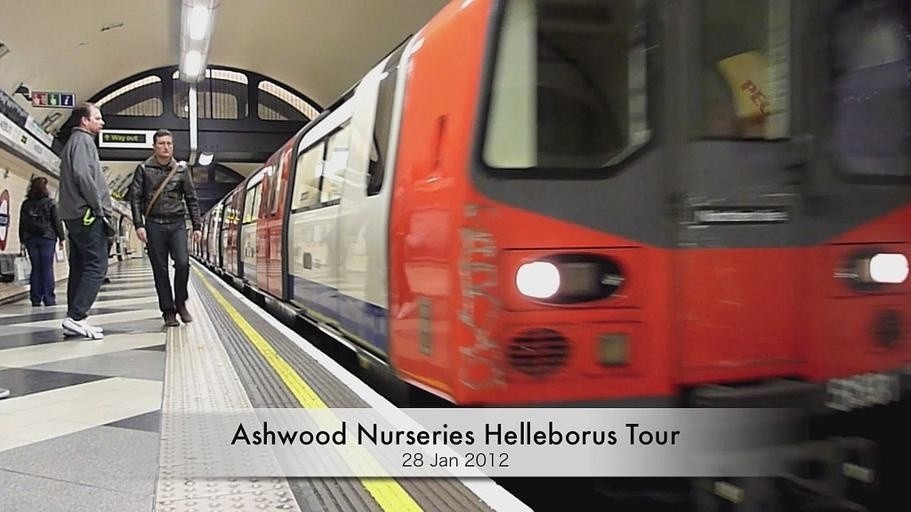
[61, 317, 104, 339]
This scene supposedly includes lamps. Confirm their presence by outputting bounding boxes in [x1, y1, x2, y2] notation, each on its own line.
[198, 152, 214, 166]
[179, 0, 217, 84]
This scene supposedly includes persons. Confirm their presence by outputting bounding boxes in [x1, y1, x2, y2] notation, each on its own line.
[130, 130, 205, 327]
[18, 176, 64, 306]
[60, 103, 116, 338]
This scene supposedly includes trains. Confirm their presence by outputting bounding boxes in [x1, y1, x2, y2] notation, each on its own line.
[188, 1, 904, 509]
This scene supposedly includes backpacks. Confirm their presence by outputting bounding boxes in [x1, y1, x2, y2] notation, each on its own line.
[19, 197, 49, 243]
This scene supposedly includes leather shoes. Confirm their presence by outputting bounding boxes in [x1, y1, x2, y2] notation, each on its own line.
[32, 302, 55, 306]
[161, 303, 193, 326]
